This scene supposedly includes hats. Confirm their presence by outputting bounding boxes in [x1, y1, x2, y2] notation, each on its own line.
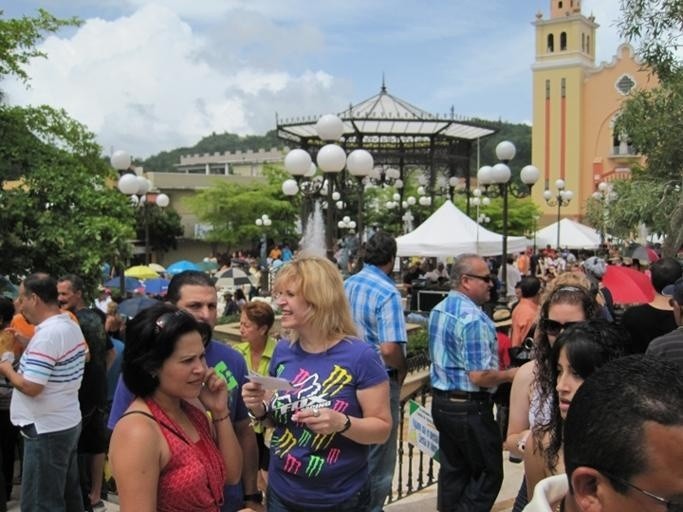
[661, 276, 683, 305]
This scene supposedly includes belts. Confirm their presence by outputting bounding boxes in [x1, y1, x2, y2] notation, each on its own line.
[434, 386, 491, 400]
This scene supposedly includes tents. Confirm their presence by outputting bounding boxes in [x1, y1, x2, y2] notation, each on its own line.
[529, 217, 625, 250]
[392, 200, 527, 283]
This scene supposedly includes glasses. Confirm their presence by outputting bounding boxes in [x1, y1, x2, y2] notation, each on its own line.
[156, 309, 191, 336]
[577, 464, 682, 512]
[463, 273, 492, 283]
[542, 319, 579, 335]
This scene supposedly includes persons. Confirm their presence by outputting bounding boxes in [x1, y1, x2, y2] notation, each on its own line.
[3, 227, 682, 512]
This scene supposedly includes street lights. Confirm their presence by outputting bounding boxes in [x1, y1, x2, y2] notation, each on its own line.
[107, 149, 169, 264]
[280, 115, 374, 272]
[370, 161, 491, 250]
[477, 140, 541, 312]
[255, 214, 273, 246]
[593, 181, 619, 258]
[542, 179, 576, 255]
